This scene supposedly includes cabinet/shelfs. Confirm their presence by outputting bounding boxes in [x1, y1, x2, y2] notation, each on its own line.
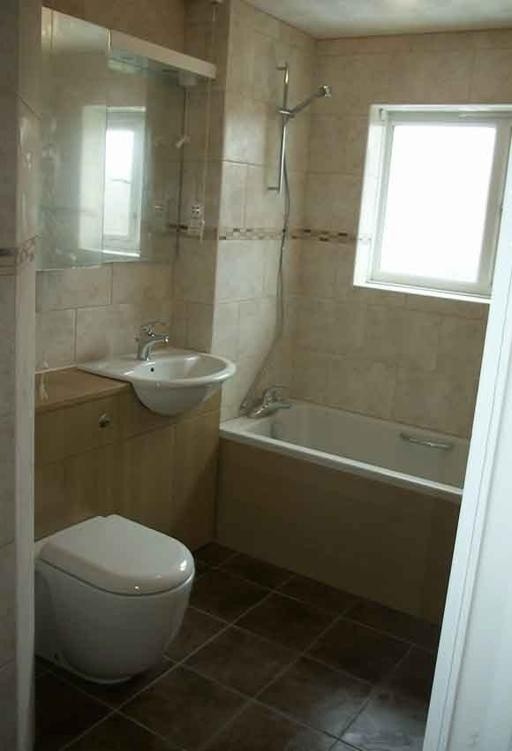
[34, 369, 221, 552]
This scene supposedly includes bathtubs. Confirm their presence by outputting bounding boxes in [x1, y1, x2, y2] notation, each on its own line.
[219, 400, 469, 505]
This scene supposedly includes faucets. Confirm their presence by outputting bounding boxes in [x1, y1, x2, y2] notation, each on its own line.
[249, 385, 291, 418]
[138, 322, 168, 359]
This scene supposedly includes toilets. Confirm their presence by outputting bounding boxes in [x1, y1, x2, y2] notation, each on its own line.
[34, 515, 197, 683]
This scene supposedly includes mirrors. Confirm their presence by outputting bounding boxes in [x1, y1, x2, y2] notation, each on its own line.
[40, 5, 190, 269]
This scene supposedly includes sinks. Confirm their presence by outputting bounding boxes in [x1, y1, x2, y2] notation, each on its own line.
[77, 349, 236, 414]
